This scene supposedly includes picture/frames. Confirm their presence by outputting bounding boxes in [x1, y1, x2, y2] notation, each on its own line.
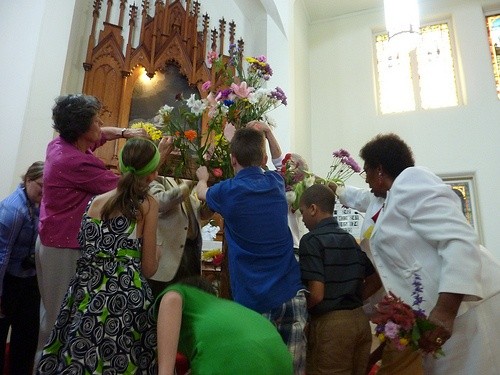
[437, 171, 484, 247]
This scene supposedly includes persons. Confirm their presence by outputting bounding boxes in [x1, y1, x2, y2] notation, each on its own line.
[35, 138, 162, 375]
[329, 133, 500, 375]
[196, 128, 310, 375]
[1, 94, 309, 375]
[299, 185, 382, 375]
[153, 278, 293, 375]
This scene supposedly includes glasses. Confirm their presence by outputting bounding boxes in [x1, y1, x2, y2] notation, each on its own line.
[359, 166, 371, 178]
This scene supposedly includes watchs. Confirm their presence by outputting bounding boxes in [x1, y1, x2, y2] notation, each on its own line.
[122, 128, 127, 138]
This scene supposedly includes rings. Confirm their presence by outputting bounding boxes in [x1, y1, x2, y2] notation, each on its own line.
[436, 338, 442, 344]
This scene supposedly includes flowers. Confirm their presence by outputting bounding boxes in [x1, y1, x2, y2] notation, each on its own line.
[200, 249, 224, 272]
[130, 44, 361, 215]
[370, 270, 446, 360]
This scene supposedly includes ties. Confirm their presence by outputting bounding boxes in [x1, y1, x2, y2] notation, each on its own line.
[177, 178, 198, 235]
